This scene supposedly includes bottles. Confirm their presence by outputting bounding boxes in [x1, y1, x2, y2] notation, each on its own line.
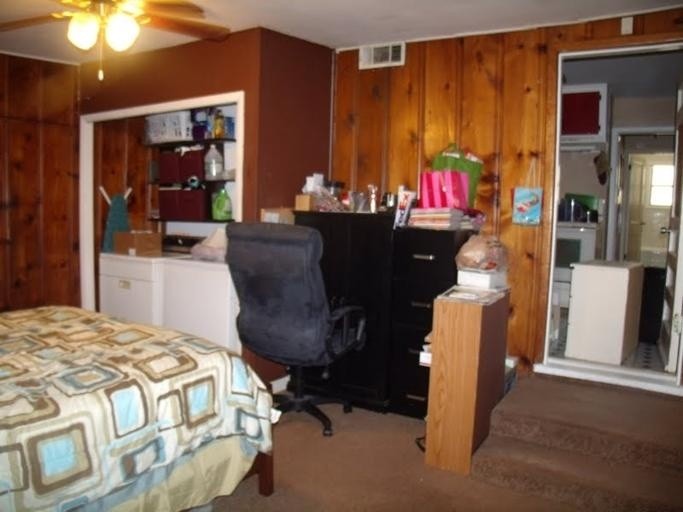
[325, 181, 345, 198]
[558, 196, 591, 224]
[214, 110, 224, 138]
[368, 185, 377, 214]
[204, 144, 223, 181]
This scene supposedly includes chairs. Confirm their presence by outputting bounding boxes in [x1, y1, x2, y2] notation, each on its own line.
[224, 220, 366, 436]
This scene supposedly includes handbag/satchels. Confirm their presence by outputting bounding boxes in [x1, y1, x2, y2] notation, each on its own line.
[417, 153, 483, 209]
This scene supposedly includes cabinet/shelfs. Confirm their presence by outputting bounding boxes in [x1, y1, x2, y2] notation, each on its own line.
[424, 283, 511, 477]
[146, 137, 235, 223]
[291, 208, 481, 420]
[98, 251, 242, 358]
[552, 220, 603, 309]
[563, 258, 645, 366]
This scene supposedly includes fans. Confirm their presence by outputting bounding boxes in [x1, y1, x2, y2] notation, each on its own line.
[0, 0, 233, 43]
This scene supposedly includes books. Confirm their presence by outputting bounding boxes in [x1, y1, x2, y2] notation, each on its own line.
[435, 267, 510, 306]
[408, 208, 480, 232]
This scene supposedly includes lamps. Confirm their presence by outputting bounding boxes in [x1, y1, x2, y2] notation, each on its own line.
[48, 0, 151, 81]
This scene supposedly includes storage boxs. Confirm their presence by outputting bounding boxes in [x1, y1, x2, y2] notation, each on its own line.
[155, 149, 209, 221]
[114, 231, 163, 257]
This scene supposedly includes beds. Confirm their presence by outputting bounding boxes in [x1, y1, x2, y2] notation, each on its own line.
[0, 304, 274, 512]
